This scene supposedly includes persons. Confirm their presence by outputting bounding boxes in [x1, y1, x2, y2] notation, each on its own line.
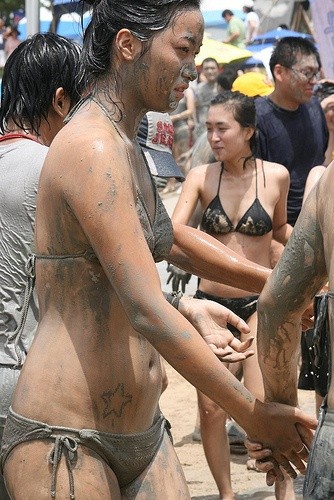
[0, 0, 334, 500]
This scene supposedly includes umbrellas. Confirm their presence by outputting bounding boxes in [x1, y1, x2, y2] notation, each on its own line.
[194, 24, 313, 67]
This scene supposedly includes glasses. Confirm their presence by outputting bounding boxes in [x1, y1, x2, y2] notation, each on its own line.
[279, 64, 322, 80]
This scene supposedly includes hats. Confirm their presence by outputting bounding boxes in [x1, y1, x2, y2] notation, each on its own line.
[231, 72, 274, 97]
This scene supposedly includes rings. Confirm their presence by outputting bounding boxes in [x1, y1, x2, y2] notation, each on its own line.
[295, 445, 307, 453]
